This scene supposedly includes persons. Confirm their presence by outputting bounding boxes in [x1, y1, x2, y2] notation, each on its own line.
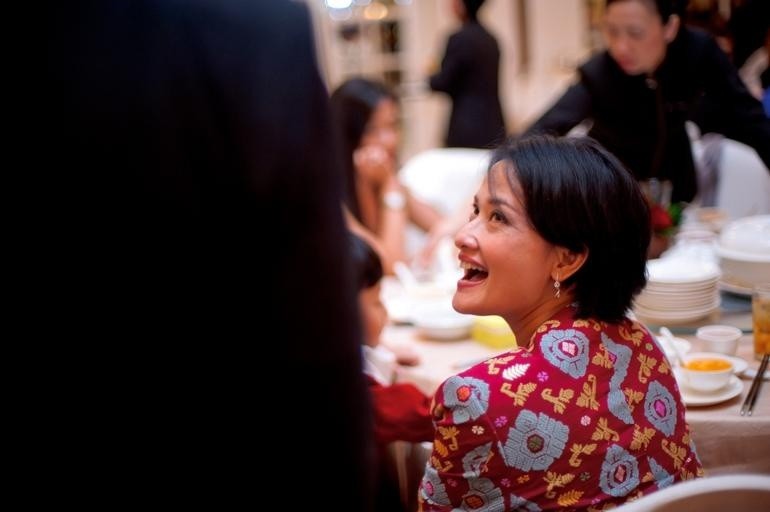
[2, 2, 408, 512]
[524, 0, 770, 205]
[349, 229, 432, 511]
[409, 134, 705, 509]
[430, 0, 508, 148]
[330, 78, 448, 278]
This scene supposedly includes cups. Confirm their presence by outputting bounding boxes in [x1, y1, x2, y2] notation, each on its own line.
[751, 283, 770, 355]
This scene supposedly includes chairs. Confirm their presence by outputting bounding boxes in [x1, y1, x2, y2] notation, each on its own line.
[610, 474, 770, 512]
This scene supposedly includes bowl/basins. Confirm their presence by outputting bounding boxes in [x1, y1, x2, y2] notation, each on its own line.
[677, 351, 735, 393]
[715, 211, 770, 290]
[656, 335, 693, 360]
[695, 324, 742, 355]
[400, 295, 476, 342]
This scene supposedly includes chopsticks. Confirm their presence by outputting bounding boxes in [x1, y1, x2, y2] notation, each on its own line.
[739, 352, 769, 417]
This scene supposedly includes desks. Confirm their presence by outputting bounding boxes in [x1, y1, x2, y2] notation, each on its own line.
[399, 314, 770, 498]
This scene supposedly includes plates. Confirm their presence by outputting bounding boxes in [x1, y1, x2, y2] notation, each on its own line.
[671, 367, 744, 408]
[725, 353, 769, 382]
[630, 251, 725, 328]
